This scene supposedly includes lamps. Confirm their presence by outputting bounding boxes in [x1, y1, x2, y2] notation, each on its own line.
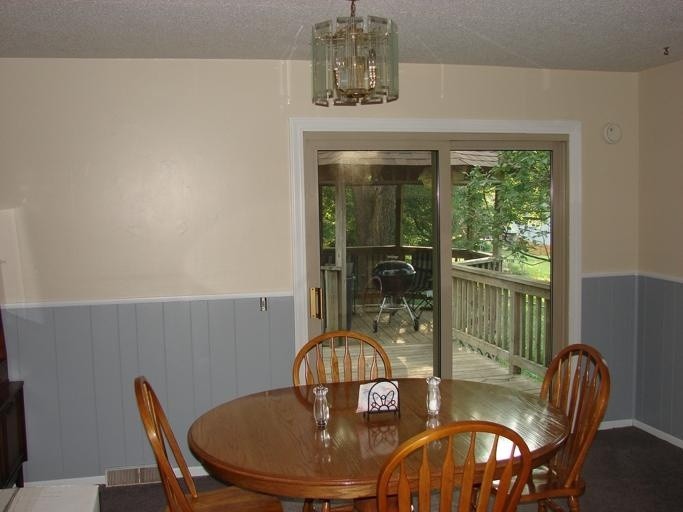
[313, 1, 399, 106]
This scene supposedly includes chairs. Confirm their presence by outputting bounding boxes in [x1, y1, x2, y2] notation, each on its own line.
[392, 250, 433, 320]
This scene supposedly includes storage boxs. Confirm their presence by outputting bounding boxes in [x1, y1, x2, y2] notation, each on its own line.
[0, 486, 100, 512]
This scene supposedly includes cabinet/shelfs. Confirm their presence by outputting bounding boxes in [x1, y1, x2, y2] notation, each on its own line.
[0, 382, 27, 489]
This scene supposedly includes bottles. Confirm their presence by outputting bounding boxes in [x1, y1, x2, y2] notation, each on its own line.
[424, 376, 441, 416]
[310, 384, 330, 426]
